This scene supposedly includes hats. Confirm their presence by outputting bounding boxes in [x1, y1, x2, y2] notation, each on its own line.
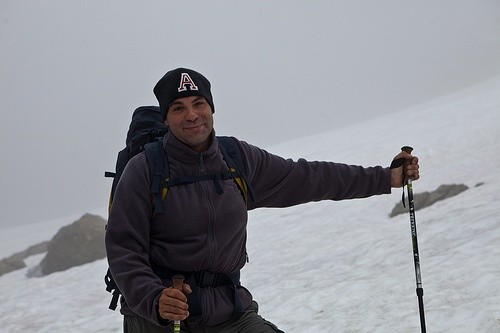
[154, 68, 214, 117]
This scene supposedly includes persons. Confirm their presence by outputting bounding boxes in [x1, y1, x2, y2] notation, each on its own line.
[105, 67, 420, 333]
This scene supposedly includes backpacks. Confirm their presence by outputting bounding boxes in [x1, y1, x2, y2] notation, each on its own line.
[103, 105, 251, 310]
